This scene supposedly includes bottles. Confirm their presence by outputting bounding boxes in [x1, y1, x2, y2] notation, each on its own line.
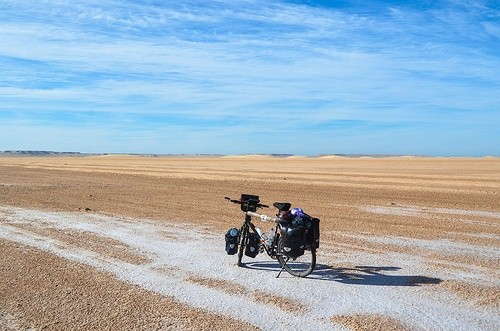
[269, 228, 274, 244]
[256, 227, 267, 241]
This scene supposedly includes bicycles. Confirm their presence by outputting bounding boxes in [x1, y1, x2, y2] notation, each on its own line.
[225, 192, 316, 280]
